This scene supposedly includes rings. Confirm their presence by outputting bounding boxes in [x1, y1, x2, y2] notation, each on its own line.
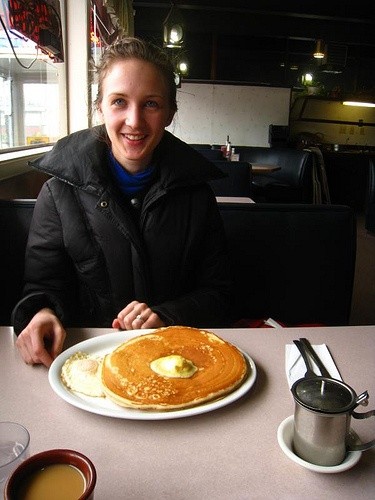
[135, 316, 145, 322]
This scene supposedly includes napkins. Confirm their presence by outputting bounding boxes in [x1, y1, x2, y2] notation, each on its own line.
[285, 344, 343, 389]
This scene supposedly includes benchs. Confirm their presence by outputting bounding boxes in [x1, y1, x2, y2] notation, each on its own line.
[0, 198, 357, 325]
[189, 144, 321, 204]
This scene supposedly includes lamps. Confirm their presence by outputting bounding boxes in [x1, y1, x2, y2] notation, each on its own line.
[162, 0, 189, 77]
[300, 38, 324, 86]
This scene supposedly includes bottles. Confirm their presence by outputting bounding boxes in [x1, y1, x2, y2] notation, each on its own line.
[225, 135, 235, 161]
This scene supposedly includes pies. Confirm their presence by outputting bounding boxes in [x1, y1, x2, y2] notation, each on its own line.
[101, 326, 247, 409]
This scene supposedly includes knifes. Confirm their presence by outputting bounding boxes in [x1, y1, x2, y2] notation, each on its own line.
[299, 338, 333, 379]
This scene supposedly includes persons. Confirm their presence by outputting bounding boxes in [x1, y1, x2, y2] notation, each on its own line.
[14, 39, 231, 367]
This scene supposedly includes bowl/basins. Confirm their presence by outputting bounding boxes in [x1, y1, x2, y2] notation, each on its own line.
[3, 448, 99, 499]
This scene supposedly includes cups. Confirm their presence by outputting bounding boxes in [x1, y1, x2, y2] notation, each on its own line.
[290, 374, 375, 467]
[0, 421, 31, 500]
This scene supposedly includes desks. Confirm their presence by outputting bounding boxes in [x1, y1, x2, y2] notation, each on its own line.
[251, 165, 281, 172]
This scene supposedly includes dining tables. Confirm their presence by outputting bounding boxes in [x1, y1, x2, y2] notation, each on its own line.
[0, 326, 375, 500]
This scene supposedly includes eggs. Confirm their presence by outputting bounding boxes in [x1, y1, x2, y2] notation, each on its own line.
[61, 352, 105, 398]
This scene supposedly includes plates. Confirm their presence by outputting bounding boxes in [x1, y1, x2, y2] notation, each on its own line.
[277, 413, 363, 474]
[47, 329, 257, 422]
